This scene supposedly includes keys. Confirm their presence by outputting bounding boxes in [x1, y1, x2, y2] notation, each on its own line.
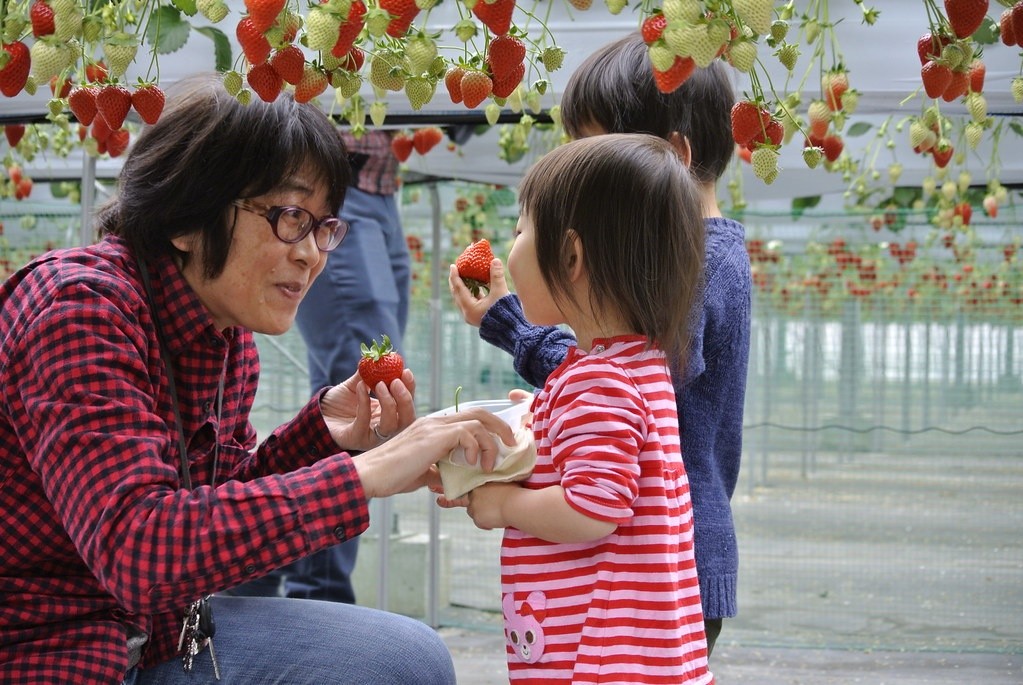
[176, 599, 224, 681]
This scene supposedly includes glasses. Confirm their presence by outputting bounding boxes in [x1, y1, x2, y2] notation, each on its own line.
[230, 196, 348, 252]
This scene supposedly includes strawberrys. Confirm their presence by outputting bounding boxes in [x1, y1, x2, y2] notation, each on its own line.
[358, 334, 402, 394]
[0, 0, 1023, 307]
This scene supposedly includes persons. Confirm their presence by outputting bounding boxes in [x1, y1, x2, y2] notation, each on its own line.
[447, 28, 754, 684]
[2, 87, 520, 683]
[283, 114, 413, 602]
[422, 131, 716, 685]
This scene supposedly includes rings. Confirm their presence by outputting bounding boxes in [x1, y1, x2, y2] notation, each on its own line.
[374, 423, 392, 440]
